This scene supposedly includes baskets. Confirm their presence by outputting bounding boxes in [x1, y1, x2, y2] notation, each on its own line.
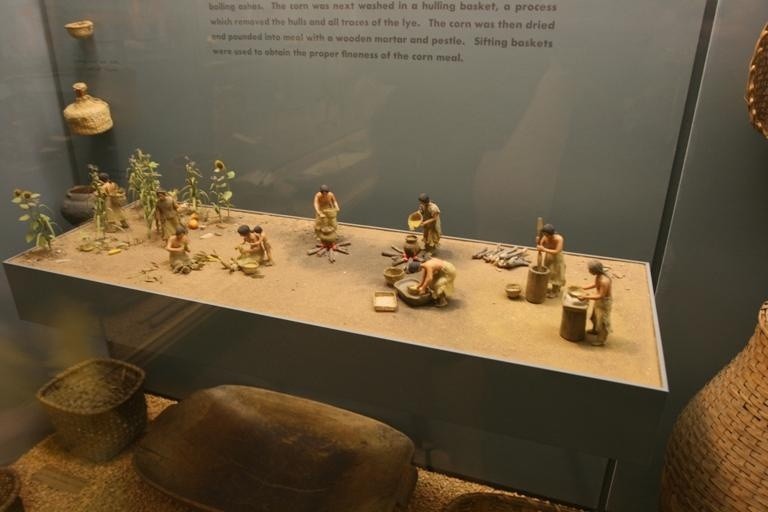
[444, 492, 561, 511]
[0, 468, 23, 512]
[661, 299, 768, 512]
[34, 356, 149, 460]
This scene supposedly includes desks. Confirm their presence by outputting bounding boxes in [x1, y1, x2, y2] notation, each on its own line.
[3, 203, 669, 417]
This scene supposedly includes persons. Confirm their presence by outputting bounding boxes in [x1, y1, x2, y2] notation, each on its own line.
[166, 229, 203, 274]
[578, 261, 612, 346]
[536, 223, 566, 298]
[418, 193, 440, 252]
[314, 185, 340, 222]
[250, 226, 273, 266]
[98, 173, 129, 233]
[408, 258, 457, 307]
[155, 191, 182, 249]
[234, 225, 265, 256]
